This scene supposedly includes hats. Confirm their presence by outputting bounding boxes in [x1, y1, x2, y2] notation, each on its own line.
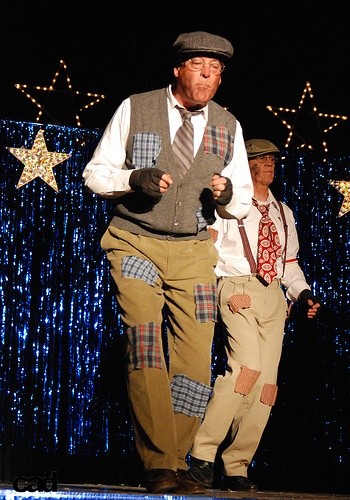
[173, 31, 234, 58]
[244, 139, 281, 158]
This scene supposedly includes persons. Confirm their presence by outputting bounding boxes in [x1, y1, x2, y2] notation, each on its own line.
[82, 31, 253, 492]
[187, 138, 321, 490]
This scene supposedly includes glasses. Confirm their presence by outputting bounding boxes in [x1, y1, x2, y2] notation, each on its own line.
[178, 58, 226, 74]
[255, 157, 275, 163]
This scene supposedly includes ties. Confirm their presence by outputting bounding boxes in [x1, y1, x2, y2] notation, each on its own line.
[171, 105, 204, 179]
[252, 198, 283, 285]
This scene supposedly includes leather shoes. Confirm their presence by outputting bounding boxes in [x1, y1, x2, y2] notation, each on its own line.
[223, 475, 258, 493]
[169, 471, 206, 493]
[189, 455, 213, 487]
[146, 469, 177, 493]
